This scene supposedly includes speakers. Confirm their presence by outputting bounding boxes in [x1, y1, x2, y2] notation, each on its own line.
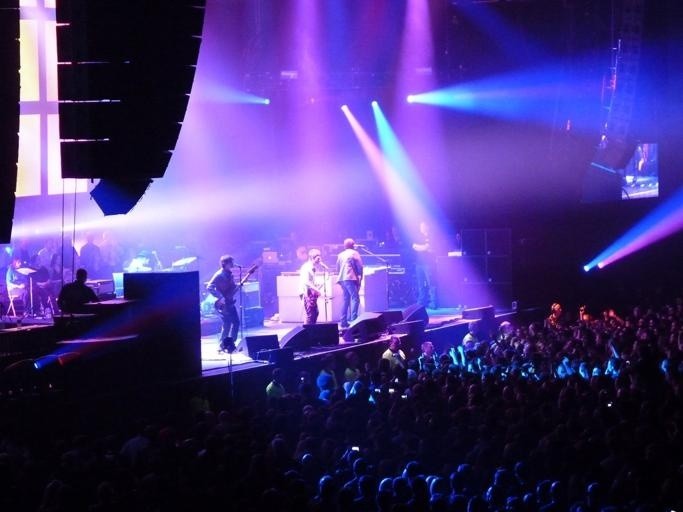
[403, 303, 429, 330]
[236, 335, 279, 357]
[372, 311, 404, 328]
[462, 306, 493, 319]
[303, 323, 339, 347]
[279, 326, 305, 351]
[123, 271, 201, 381]
[344, 312, 385, 343]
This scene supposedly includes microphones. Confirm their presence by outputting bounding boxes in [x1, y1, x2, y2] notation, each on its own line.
[353, 244, 365, 249]
[319, 262, 328, 270]
[233, 263, 242, 268]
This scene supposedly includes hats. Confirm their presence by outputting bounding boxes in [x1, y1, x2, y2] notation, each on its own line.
[344, 239, 355, 248]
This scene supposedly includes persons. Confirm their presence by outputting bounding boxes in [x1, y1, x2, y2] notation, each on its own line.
[336, 238, 364, 327]
[0, 303, 683, 512]
[9, 229, 120, 318]
[412, 222, 440, 310]
[298, 248, 323, 325]
[207, 254, 240, 351]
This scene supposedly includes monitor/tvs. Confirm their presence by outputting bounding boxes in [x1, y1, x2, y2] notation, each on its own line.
[621, 142, 659, 200]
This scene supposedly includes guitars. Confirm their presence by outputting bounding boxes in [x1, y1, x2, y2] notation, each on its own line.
[215, 264, 258, 318]
[303, 281, 325, 308]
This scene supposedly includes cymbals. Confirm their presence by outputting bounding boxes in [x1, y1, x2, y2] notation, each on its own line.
[173, 257, 197, 267]
